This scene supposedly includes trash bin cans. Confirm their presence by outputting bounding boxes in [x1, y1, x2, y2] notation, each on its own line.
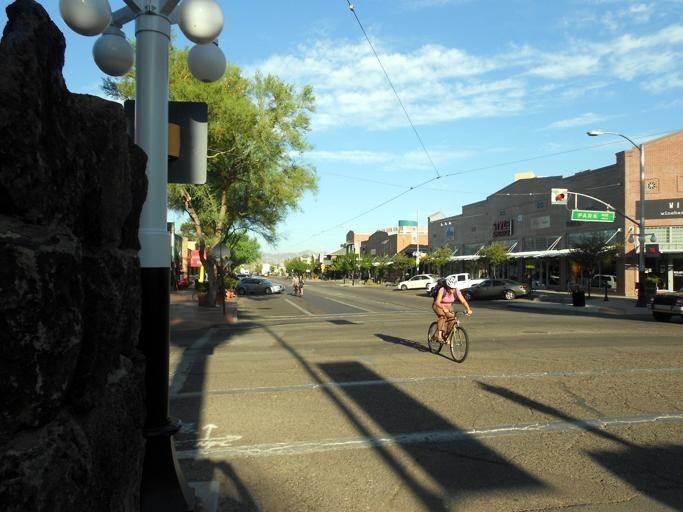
[371, 278, 375, 283]
[572, 288, 585, 307]
[638, 281, 656, 307]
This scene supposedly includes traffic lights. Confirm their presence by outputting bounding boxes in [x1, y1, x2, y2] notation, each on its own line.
[646, 244, 659, 258]
[550, 188, 568, 205]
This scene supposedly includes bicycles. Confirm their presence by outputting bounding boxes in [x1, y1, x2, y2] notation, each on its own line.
[428, 309, 469, 363]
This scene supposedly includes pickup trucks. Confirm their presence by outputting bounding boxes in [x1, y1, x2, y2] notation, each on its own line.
[426, 273, 490, 296]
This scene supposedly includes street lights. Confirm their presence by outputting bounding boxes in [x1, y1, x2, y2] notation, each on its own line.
[59, 0, 226, 449]
[587, 131, 656, 307]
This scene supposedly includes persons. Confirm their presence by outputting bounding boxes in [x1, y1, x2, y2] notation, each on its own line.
[299, 275, 305, 295]
[432, 276, 473, 344]
[291, 275, 298, 295]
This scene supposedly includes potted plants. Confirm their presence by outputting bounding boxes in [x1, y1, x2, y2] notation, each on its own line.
[191, 279, 208, 306]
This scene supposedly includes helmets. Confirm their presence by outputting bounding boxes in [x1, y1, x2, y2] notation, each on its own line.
[445, 275, 457, 288]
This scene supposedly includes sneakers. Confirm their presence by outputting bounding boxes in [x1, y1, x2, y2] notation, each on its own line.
[436, 338, 446, 344]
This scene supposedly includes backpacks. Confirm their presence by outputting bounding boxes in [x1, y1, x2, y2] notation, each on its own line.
[430, 278, 445, 298]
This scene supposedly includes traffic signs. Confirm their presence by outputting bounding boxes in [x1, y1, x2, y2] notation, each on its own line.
[571, 209, 615, 223]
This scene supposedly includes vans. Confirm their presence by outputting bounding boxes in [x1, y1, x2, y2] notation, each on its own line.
[593, 274, 617, 288]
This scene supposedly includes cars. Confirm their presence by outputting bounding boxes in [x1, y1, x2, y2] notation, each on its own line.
[460, 280, 530, 301]
[647, 287, 683, 322]
[235, 277, 282, 294]
[397, 273, 442, 291]
